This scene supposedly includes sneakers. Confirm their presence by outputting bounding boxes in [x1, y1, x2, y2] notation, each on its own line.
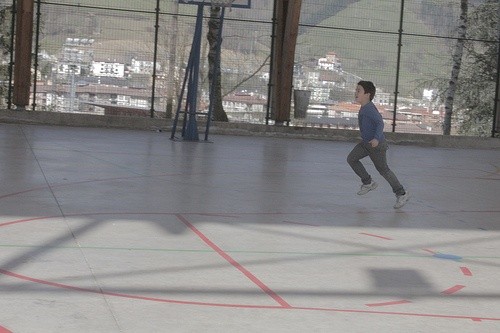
[393, 190, 411, 208]
[358, 181, 377, 195]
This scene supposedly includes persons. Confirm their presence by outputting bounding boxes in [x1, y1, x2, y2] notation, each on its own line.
[346, 80, 411, 209]
